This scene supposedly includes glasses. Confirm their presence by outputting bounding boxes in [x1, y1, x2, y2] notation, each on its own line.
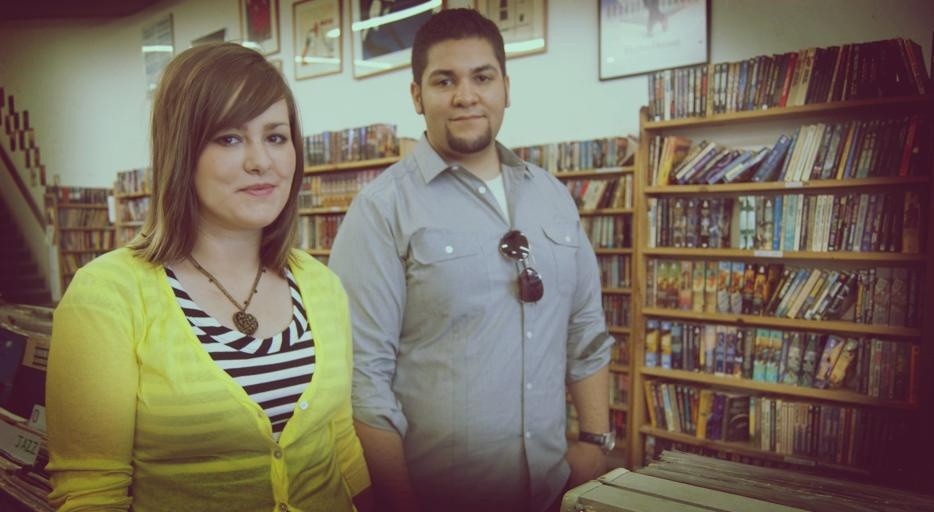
[498, 230, 543, 304]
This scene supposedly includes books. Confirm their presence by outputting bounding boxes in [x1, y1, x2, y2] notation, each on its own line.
[54, 165, 153, 286]
[296, 126, 403, 261]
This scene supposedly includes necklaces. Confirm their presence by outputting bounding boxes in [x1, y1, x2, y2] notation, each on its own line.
[186, 254, 268, 336]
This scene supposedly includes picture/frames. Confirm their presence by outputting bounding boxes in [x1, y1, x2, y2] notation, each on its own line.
[234, 0, 281, 58]
[472, 0, 550, 61]
[286, 0, 344, 80]
[345, 0, 448, 82]
[597, 0, 712, 82]
[268, 54, 286, 85]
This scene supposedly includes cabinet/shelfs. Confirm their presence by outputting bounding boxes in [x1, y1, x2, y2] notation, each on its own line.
[114, 178, 148, 255]
[548, 165, 637, 456]
[292, 137, 419, 269]
[59, 201, 114, 291]
[628, 74, 933, 487]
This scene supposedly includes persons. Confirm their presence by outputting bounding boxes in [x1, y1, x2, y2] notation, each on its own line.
[44, 37, 380, 512]
[326, 1, 614, 512]
